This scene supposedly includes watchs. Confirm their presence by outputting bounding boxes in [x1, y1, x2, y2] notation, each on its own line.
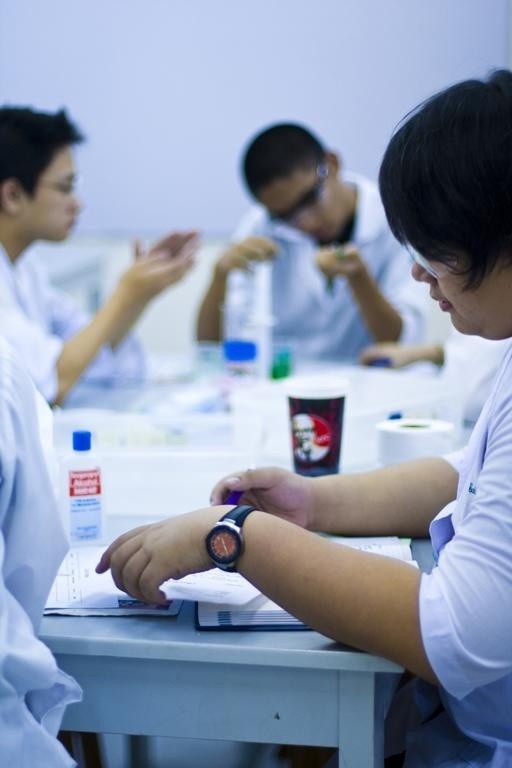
[205, 502, 254, 573]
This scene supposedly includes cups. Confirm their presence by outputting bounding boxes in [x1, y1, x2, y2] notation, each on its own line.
[285, 394, 346, 477]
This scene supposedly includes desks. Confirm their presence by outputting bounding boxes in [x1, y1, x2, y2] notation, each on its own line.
[28, 541, 437, 767]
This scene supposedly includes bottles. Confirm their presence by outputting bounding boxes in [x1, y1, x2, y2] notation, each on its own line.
[63, 430, 103, 538]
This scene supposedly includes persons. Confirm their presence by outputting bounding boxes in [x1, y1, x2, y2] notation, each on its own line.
[1, 350, 87, 768]
[89, 64, 511, 768]
[357, 341, 455, 370]
[195, 120, 455, 370]
[0, 102, 201, 415]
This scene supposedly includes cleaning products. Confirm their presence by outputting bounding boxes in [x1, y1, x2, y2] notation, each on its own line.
[60, 431, 101, 551]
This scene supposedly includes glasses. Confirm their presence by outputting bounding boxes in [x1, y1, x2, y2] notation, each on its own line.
[402, 238, 458, 278]
[268, 165, 328, 224]
